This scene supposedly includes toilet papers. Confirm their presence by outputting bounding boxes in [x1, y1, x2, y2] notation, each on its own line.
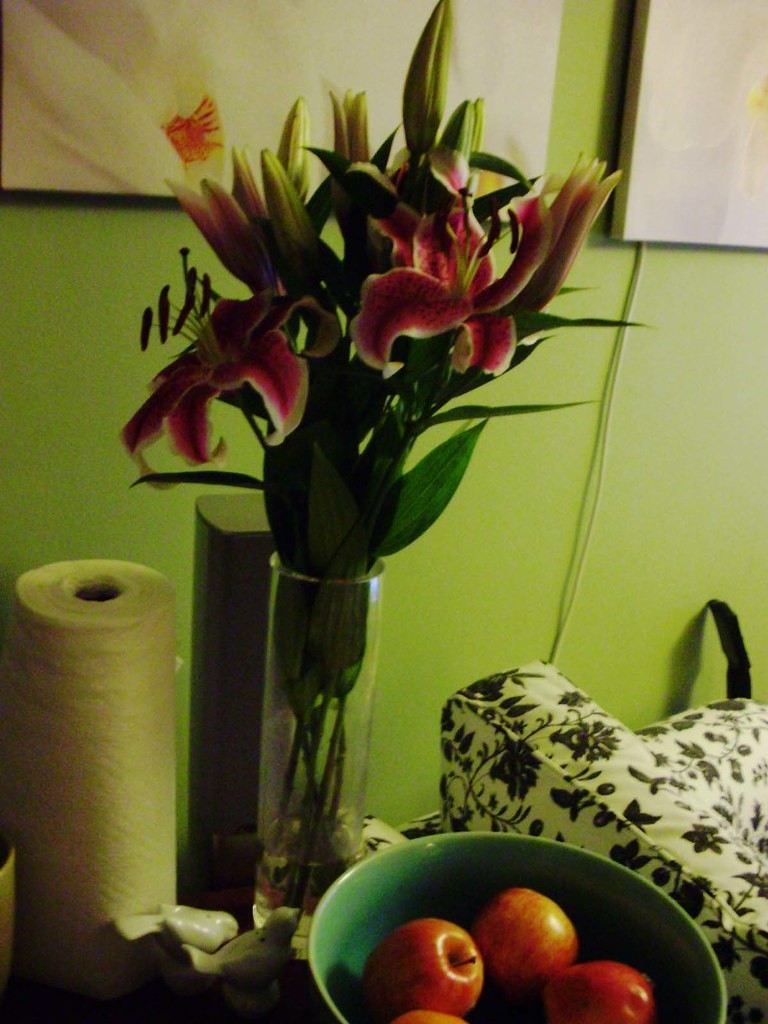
[0, 558, 179, 1006]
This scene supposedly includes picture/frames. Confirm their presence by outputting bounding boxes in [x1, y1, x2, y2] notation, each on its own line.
[609, 0, 768, 250]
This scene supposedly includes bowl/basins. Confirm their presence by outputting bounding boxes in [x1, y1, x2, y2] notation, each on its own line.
[307, 831, 727, 1024]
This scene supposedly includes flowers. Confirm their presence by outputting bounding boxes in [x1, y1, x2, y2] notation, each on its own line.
[120, 0, 645, 922]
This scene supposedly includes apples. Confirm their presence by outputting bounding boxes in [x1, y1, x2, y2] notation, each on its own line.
[544, 961, 653, 1024]
[471, 887, 578, 1001]
[364, 917, 484, 1017]
[389, 1009, 468, 1024]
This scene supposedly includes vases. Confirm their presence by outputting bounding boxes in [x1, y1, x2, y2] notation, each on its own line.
[251, 555, 386, 959]
